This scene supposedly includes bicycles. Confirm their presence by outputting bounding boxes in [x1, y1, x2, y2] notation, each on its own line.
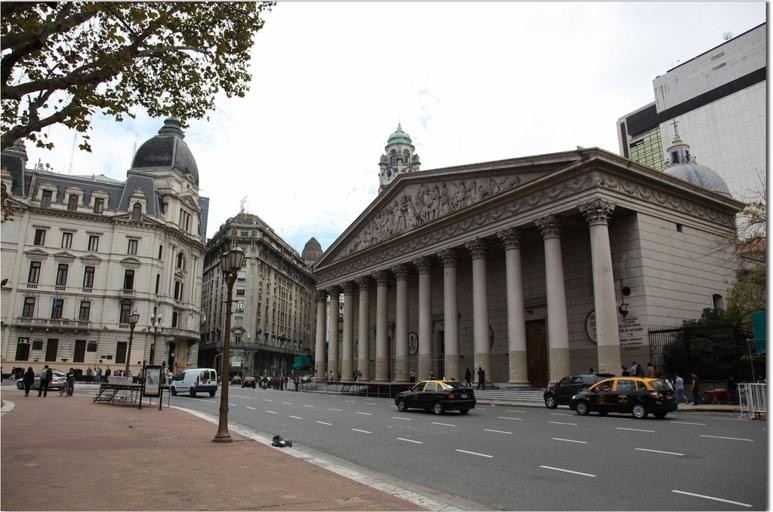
[58, 379, 73, 399]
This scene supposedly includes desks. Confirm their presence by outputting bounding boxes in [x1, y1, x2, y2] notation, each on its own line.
[703, 387, 729, 405]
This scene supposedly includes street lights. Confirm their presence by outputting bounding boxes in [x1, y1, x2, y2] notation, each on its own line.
[213, 241, 246, 443]
[148, 312, 165, 365]
[125, 308, 141, 377]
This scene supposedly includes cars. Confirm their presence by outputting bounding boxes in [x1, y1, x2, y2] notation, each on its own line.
[241, 376, 256, 389]
[543, 374, 616, 409]
[569, 377, 678, 419]
[16, 370, 67, 390]
[231, 376, 241, 385]
[395, 380, 476, 415]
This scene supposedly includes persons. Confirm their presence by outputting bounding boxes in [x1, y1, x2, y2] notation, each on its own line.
[476, 366, 485, 390]
[258, 372, 313, 392]
[36, 364, 49, 398]
[726, 373, 738, 404]
[670, 373, 690, 405]
[329, 370, 333, 380]
[65, 367, 76, 398]
[428, 369, 434, 379]
[323, 368, 328, 379]
[351, 368, 357, 382]
[588, 366, 593, 372]
[738, 384, 747, 406]
[408, 368, 416, 383]
[464, 367, 472, 388]
[0, 360, 180, 385]
[355, 369, 362, 381]
[690, 370, 704, 405]
[620, 360, 658, 378]
[21, 366, 33, 397]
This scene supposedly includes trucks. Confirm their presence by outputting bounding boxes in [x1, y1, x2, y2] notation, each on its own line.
[169, 367, 218, 398]
